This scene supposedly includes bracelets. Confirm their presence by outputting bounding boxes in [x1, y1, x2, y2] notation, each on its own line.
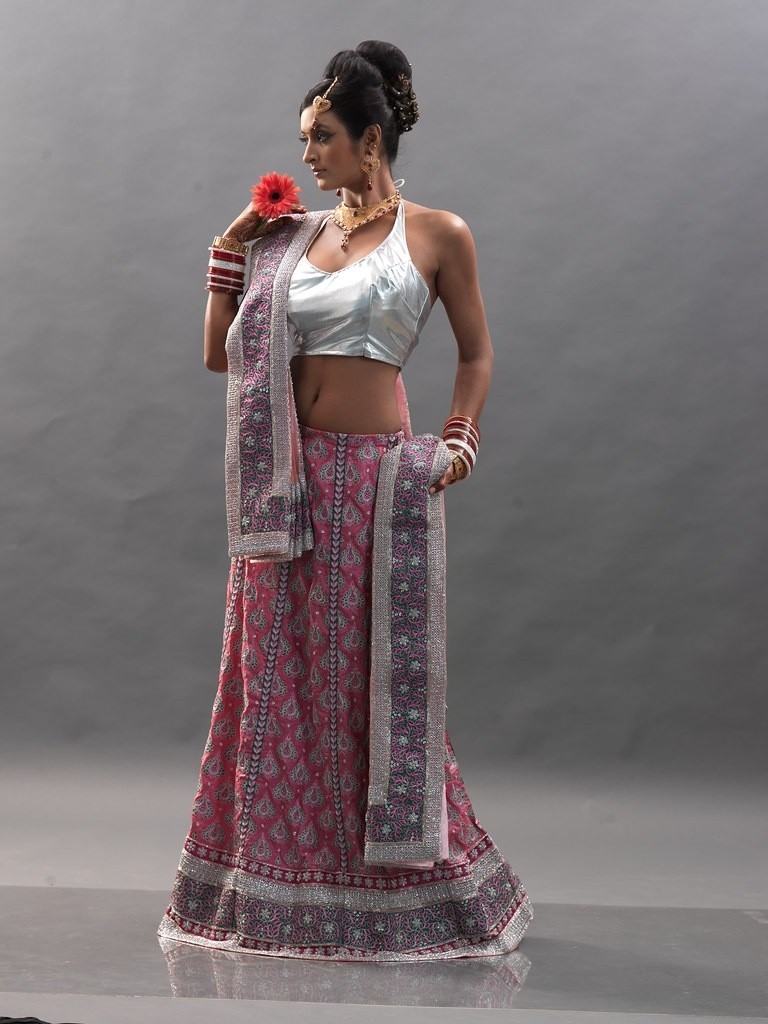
[205, 234, 250, 296]
[440, 414, 481, 484]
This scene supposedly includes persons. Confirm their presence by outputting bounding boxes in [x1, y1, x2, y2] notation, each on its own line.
[154, 38, 534, 964]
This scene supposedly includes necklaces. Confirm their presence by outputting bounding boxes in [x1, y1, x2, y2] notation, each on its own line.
[331, 190, 401, 252]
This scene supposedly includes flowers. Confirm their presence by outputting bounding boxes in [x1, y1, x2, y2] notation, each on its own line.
[250, 170, 302, 220]
